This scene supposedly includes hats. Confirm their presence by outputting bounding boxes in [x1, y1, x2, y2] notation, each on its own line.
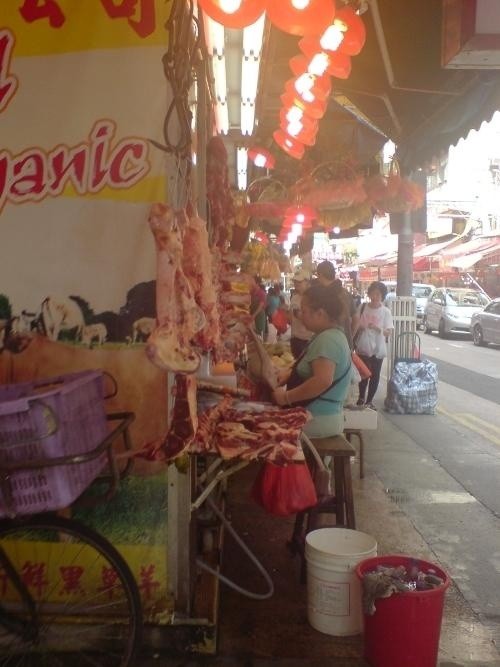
[290, 271, 311, 282]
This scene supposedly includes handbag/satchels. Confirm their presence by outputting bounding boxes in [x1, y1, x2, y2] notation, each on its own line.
[283, 368, 320, 408]
[384, 360, 439, 414]
[350, 352, 371, 384]
[250, 460, 317, 516]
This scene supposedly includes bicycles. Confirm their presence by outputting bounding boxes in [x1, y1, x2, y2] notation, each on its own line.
[1, 412, 138, 665]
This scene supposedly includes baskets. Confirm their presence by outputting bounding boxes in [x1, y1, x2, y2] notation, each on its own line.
[1, 368, 109, 519]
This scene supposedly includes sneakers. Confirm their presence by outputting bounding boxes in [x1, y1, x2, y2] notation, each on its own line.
[357, 398, 377, 410]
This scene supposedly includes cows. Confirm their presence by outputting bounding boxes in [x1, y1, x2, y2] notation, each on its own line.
[132, 317, 157, 344]
[32, 295, 108, 350]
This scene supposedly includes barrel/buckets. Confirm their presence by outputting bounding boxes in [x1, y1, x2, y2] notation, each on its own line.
[304, 527, 378, 637]
[355, 554, 450, 667]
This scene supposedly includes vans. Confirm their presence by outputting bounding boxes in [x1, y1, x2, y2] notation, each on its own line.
[383, 282, 433, 324]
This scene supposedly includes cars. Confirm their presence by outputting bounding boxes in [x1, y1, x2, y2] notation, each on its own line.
[470, 298, 500, 347]
[422, 287, 491, 338]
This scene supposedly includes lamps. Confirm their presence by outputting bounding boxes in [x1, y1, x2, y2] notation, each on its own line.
[197, 0, 269, 31]
[244, 142, 278, 173]
[244, 197, 321, 249]
[268, 0, 366, 161]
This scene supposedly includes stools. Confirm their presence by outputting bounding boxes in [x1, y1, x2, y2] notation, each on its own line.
[290, 429, 364, 584]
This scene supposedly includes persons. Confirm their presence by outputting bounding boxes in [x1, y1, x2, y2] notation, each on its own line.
[244, 261, 394, 448]
